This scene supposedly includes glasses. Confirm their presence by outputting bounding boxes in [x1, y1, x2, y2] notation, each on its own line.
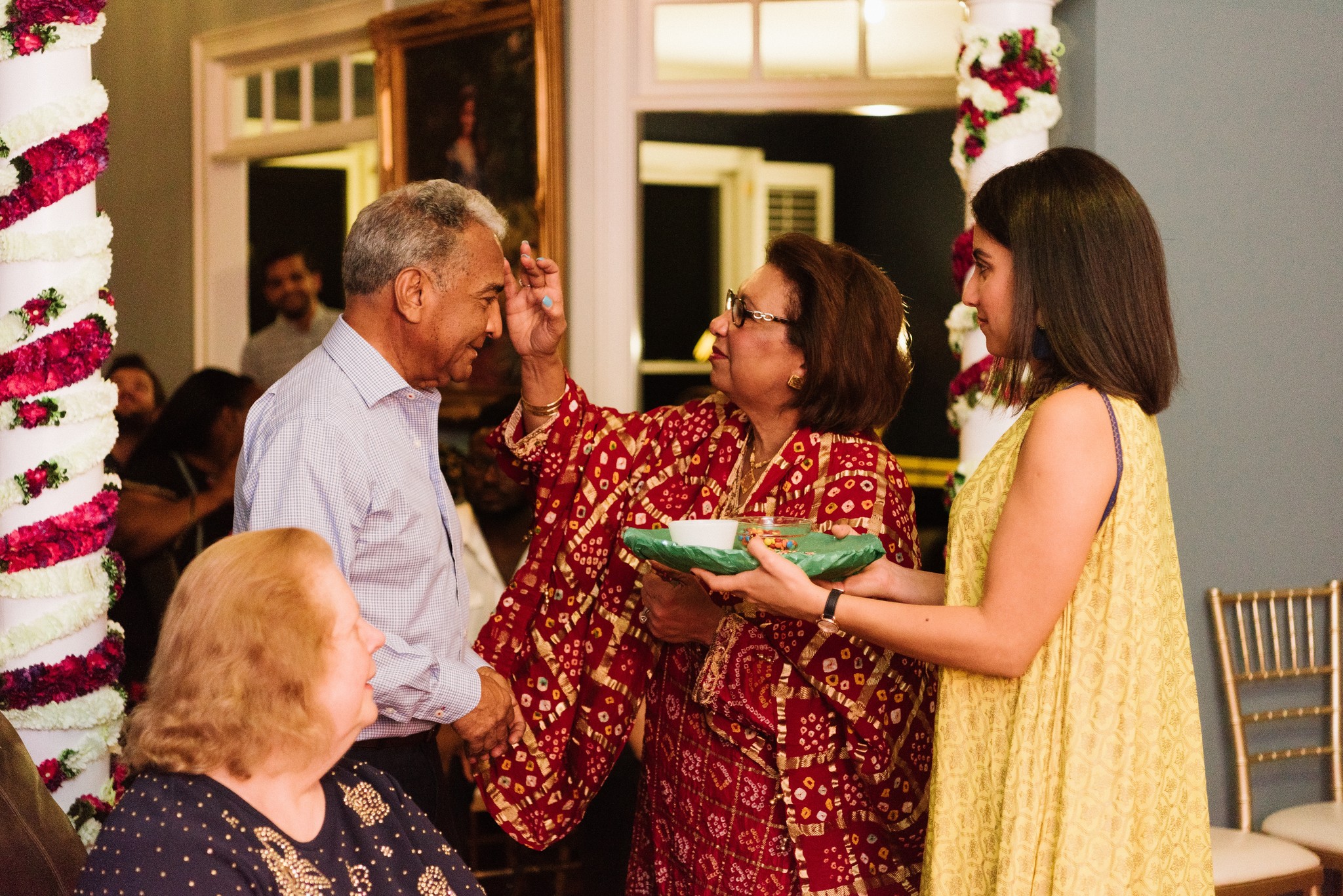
[724, 288, 795, 327]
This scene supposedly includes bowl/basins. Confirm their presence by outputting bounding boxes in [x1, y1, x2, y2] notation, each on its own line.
[731, 514, 812, 552]
[666, 516, 737, 554]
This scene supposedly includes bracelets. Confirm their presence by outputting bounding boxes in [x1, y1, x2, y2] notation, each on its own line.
[517, 384, 566, 417]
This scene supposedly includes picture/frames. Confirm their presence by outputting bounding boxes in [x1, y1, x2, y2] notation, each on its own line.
[358, 2, 572, 427]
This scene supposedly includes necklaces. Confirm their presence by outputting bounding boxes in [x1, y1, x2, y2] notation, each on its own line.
[739, 442, 774, 497]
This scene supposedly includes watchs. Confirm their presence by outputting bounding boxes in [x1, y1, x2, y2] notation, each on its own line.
[811, 587, 845, 634]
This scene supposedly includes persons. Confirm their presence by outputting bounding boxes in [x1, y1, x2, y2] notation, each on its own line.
[69, 526, 488, 896]
[230, 183, 507, 872]
[237, 243, 341, 388]
[460, 230, 942, 896]
[680, 145, 1219, 896]
[111, 364, 264, 683]
[103, 350, 165, 471]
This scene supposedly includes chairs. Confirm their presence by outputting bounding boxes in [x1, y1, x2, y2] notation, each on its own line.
[1206, 580, 1343, 896]
[1208, 825, 1327, 896]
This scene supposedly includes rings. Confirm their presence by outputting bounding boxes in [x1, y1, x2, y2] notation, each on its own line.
[518, 279, 532, 289]
